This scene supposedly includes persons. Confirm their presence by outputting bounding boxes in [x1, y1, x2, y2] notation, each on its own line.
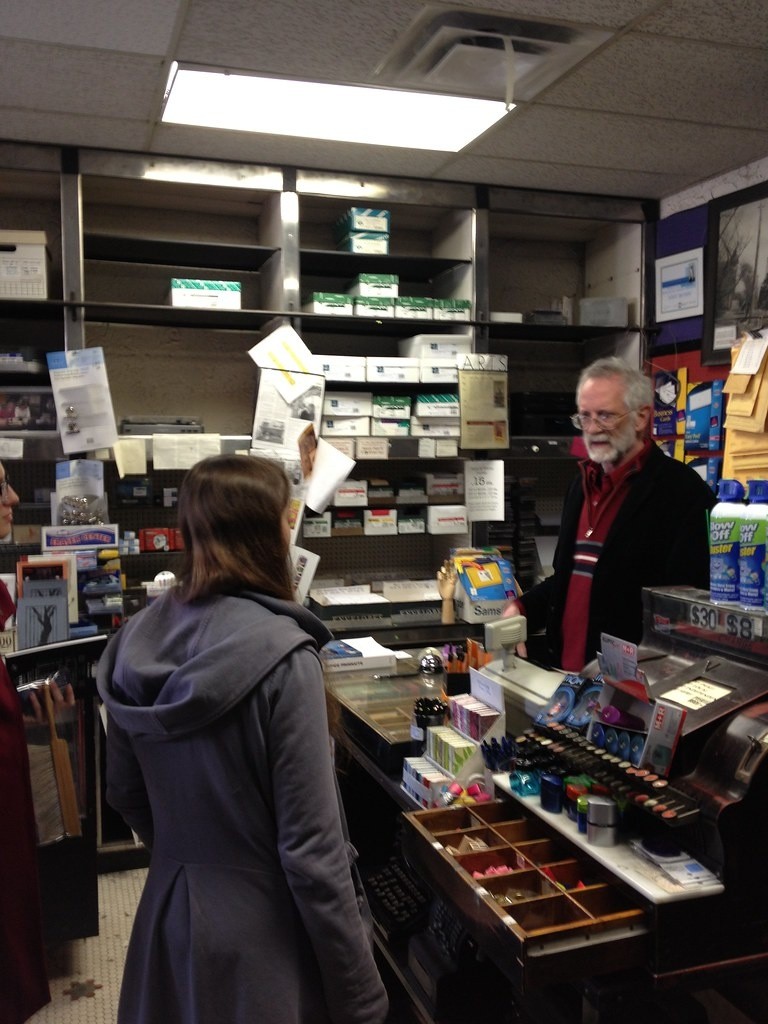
[499, 356, 717, 673]
[94, 448, 394, 1024]
[0, 459, 76, 1024]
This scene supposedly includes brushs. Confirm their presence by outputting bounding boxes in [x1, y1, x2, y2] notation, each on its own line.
[26, 683, 83, 846]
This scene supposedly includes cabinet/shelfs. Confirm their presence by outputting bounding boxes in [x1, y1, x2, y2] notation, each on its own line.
[0, 139, 662, 631]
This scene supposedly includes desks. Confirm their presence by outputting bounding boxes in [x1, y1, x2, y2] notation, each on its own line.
[324, 648, 445, 745]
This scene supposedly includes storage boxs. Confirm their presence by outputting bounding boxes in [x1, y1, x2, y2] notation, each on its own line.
[162, 277, 241, 309]
[0, 229, 48, 299]
[302, 505, 469, 538]
[329, 465, 465, 507]
[300, 272, 472, 322]
[334, 206, 390, 256]
[319, 391, 461, 461]
[0, 386, 56, 431]
[311, 333, 471, 384]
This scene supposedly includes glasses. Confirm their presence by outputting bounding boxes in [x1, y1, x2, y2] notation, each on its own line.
[0, 475, 12, 505]
[569, 410, 633, 432]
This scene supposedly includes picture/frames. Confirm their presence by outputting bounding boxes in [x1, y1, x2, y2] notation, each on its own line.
[16, 561, 70, 650]
[700, 180, 768, 367]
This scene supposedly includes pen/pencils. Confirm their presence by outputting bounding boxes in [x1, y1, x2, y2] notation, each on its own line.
[373, 672, 421, 680]
